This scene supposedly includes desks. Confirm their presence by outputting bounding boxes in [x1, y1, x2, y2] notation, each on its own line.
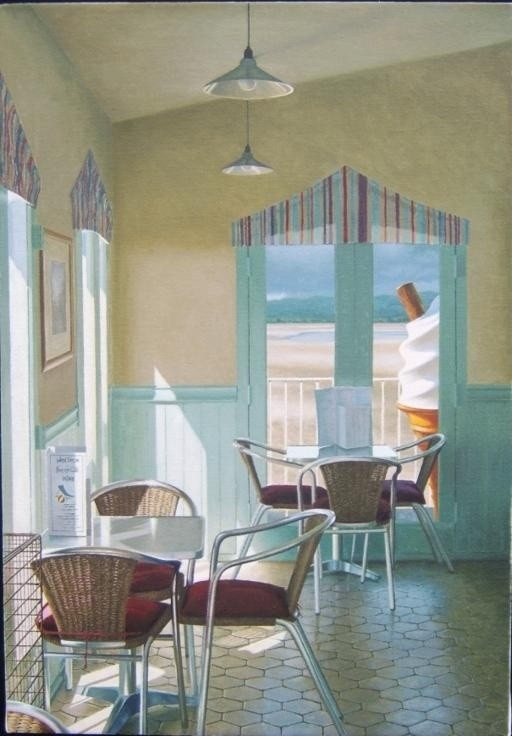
[285, 441, 396, 593]
[32, 511, 211, 710]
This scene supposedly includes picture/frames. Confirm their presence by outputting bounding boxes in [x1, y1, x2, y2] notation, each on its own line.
[37, 228, 75, 371]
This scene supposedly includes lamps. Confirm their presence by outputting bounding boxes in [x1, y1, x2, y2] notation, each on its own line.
[201, 0, 295, 178]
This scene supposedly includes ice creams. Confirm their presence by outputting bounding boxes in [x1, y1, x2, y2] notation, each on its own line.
[395, 282, 440, 520]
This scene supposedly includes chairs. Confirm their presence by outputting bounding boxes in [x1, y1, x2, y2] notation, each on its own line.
[349, 432, 455, 583]
[291, 454, 404, 615]
[234, 435, 333, 615]
[177, 505, 343, 734]
[81, 480, 200, 700]
[32, 545, 191, 734]
